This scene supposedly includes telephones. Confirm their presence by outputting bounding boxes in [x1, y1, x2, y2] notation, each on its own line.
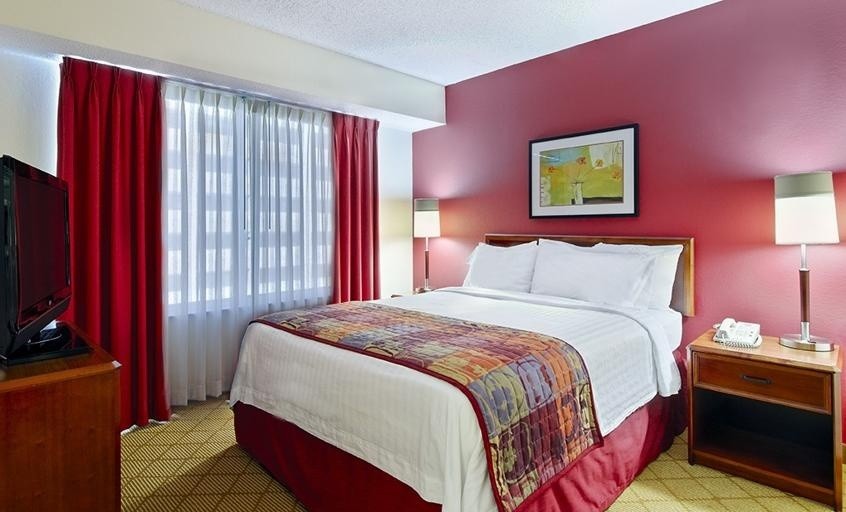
[712, 317, 761, 345]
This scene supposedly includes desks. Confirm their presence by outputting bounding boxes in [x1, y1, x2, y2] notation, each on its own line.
[0, 320, 125, 510]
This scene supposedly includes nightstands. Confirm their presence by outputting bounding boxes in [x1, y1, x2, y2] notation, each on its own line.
[684, 323, 845, 512]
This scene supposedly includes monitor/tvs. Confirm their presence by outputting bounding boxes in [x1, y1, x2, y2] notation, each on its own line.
[1, 154, 92, 369]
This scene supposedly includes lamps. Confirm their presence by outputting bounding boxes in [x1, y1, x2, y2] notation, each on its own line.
[772, 169, 840, 352]
[412, 198, 440, 294]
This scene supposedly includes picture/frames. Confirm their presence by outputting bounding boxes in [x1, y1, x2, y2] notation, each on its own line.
[529, 121, 639, 218]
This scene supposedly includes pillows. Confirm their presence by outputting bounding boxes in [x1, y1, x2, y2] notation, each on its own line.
[463, 237, 684, 312]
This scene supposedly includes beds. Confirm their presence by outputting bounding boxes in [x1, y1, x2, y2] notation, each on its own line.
[233, 233, 696, 512]
